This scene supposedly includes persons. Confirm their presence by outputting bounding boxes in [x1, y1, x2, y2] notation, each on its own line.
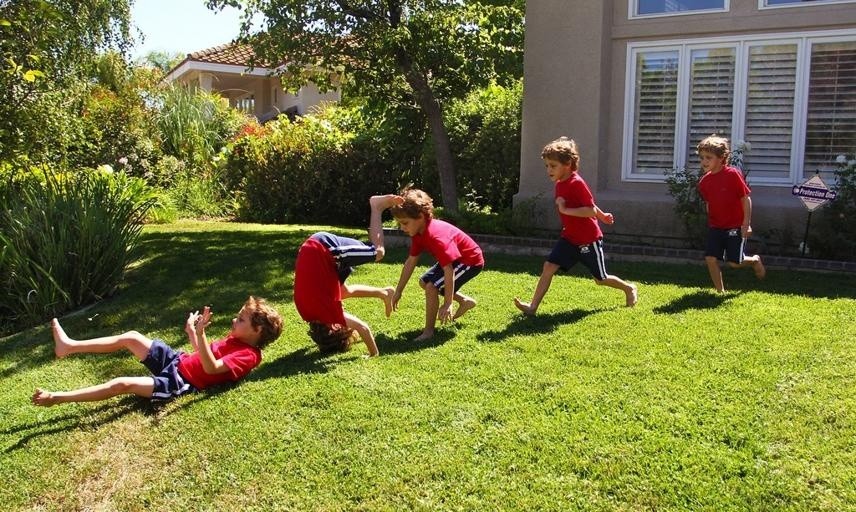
[294, 195, 405, 359]
[513, 136, 637, 315]
[389, 187, 485, 342]
[694, 134, 765, 294]
[31, 296, 282, 406]
